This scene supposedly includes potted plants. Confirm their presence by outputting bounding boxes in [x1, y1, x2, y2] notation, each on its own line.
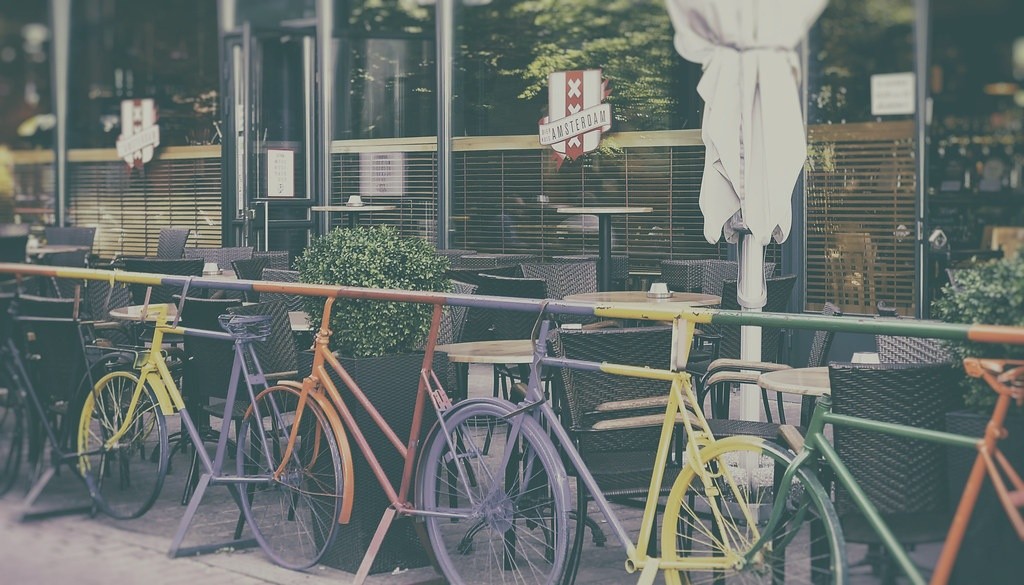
[284, 228, 448, 578]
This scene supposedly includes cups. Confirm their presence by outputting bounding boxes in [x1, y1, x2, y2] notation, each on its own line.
[348, 196, 361, 203]
[204, 262, 219, 271]
[851, 351, 880, 364]
[649, 283, 670, 292]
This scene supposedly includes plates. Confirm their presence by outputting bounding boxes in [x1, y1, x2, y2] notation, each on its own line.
[646, 292, 673, 298]
[202, 271, 223, 274]
[345, 202, 365, 206]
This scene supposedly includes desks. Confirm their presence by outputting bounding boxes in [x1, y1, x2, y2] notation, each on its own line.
[107, 300, 263, 474]
[433, 339, 610, 557]
[560, 291, 723, 328]
[758, 366, 833, 585]
[23, 243, 91, 298]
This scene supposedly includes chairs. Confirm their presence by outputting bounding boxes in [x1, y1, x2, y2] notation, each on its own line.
[0, 224, 966, 585]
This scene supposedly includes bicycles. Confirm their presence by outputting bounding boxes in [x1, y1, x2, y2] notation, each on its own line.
[0, 284, 1023, 585]
